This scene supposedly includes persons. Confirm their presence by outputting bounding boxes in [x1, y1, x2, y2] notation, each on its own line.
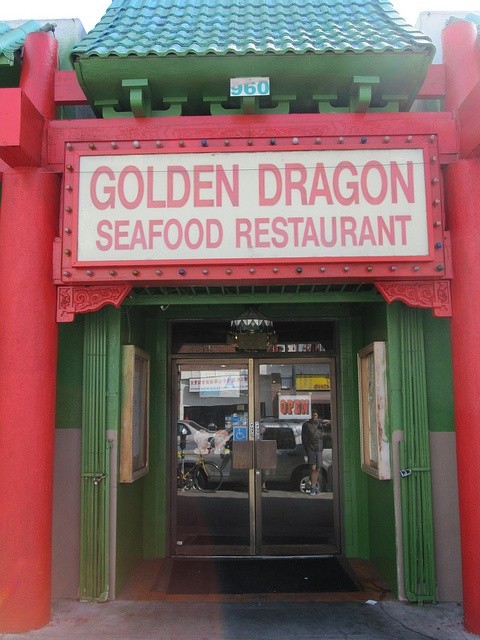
[302, 409, 328, 496]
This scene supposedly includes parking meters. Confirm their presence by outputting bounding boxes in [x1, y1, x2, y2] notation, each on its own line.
[181, 430, 187, 491]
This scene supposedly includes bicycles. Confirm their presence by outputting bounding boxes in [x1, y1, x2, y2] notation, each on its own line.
[175, 448, 224, 494]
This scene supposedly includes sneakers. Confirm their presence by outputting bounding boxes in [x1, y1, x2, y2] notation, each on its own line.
[310, 486, 317, 495]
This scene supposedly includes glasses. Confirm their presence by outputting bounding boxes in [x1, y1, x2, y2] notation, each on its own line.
[311, 414, 317, 417]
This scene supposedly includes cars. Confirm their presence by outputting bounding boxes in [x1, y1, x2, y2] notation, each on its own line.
[178, 419, 223, 453]
[198, 420, 333, 494]
